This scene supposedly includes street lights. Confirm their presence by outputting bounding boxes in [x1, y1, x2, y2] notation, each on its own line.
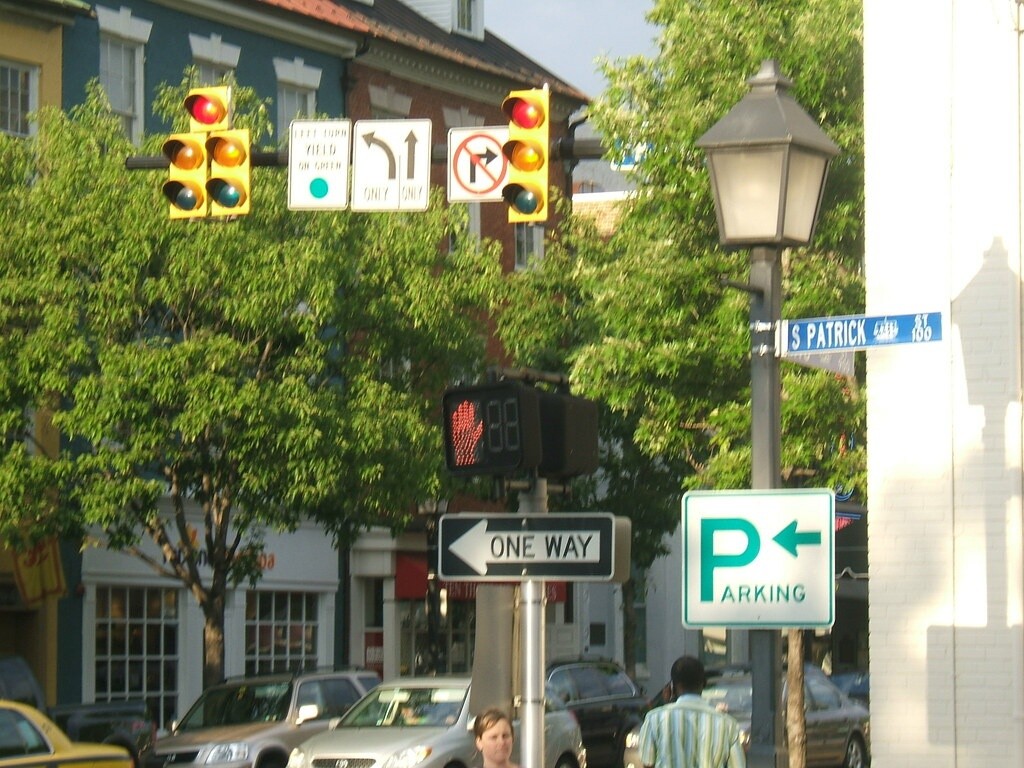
[689, 52, 846, 768]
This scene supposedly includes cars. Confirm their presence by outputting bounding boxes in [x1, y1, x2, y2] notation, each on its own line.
[696, 665, 871, 768]
[286, 672, 587, 768]
[0, 700, 136, 768]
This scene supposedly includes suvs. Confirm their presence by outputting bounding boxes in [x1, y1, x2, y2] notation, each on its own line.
[545, 652, 646, 768]
[138, 666, 381, 768]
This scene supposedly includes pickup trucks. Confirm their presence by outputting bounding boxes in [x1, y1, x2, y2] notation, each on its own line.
[0, 652, 151, 760]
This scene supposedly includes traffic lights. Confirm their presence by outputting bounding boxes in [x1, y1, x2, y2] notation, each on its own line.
[543, 392, 600, 483]
[501, 88, 548, 222]
[184, 87, 233, 131]
[441, 382, 539, 479]
[163, 133, 208, 218]
[205, 130, 251, 217]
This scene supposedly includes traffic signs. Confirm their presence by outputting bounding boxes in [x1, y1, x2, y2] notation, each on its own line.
[437, 514, 617, 583]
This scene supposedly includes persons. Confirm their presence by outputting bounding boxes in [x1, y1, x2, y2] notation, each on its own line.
[436, 701, 463, 725]
[641, 656, 746, 768]
[474, 709, 521, 768]
[383, 691, 426, 725]
